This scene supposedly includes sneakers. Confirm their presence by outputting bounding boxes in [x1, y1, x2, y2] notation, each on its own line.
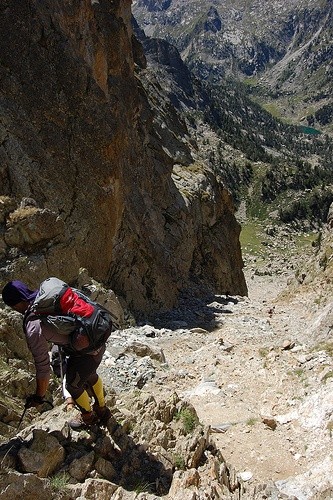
[67, 417, 99, 432]
[92, 407, 112, 424]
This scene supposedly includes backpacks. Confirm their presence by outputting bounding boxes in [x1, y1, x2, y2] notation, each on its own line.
[23, 277, 117, 357]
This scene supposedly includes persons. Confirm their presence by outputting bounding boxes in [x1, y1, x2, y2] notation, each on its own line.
[2, 281, 114, 432]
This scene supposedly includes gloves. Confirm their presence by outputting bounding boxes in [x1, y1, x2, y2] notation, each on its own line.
[24, 394, 44, 409]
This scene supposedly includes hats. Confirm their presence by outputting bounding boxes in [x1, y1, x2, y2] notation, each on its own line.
[2, 281, 39, 307]
